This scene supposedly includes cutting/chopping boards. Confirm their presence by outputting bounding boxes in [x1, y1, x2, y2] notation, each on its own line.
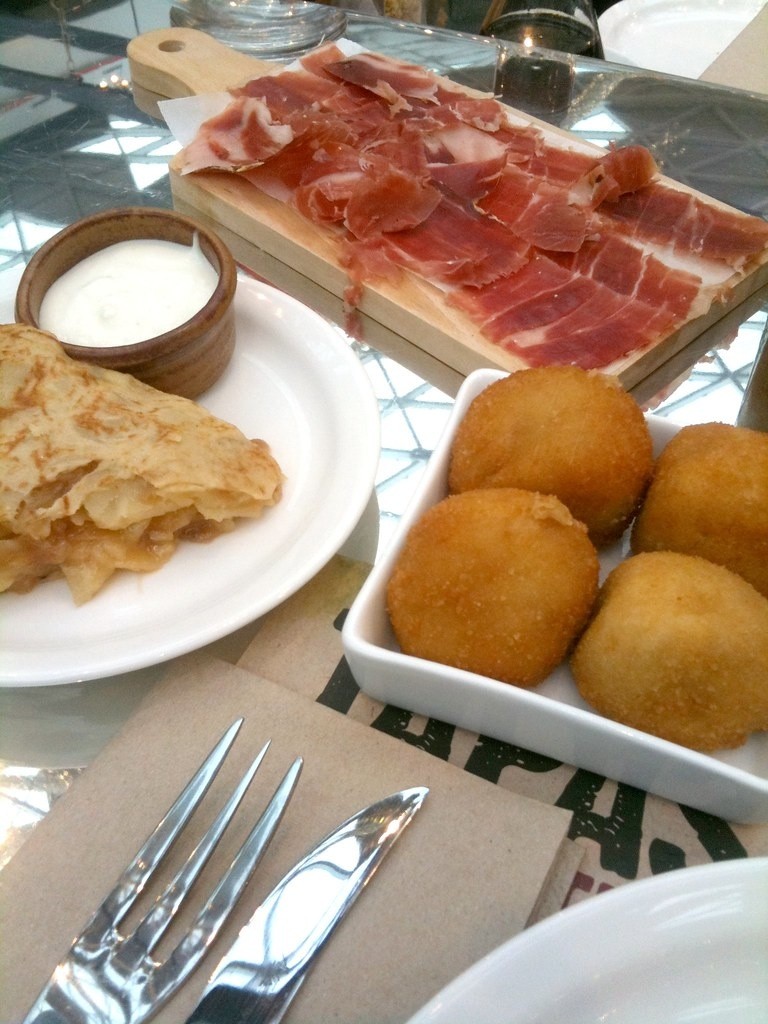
[126, 28, 768, 393]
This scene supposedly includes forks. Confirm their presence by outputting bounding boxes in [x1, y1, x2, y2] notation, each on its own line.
[24, 717, 303, 1024]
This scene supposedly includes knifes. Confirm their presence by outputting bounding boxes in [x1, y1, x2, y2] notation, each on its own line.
[186, 786, 429, 1024]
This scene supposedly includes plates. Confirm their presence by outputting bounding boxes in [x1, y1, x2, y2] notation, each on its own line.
[343, 367, 768, 827]
[0, 272, 382, 686]
[405, 856, 768, 1024]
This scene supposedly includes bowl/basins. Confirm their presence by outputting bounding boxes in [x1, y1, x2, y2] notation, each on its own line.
[17, 207, 238, 401]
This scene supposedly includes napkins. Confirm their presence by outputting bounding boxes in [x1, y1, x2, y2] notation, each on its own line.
[0, 650, 584, 1024]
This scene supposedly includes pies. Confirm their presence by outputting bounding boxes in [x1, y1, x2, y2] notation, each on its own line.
[0, 321, 288, 606]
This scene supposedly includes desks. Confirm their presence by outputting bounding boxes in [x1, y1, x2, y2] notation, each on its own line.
[0, 0, 768, 1024]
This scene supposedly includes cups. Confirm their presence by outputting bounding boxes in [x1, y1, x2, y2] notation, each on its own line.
[170, 0, 349, 61]
[477, 17, 597, 129]
